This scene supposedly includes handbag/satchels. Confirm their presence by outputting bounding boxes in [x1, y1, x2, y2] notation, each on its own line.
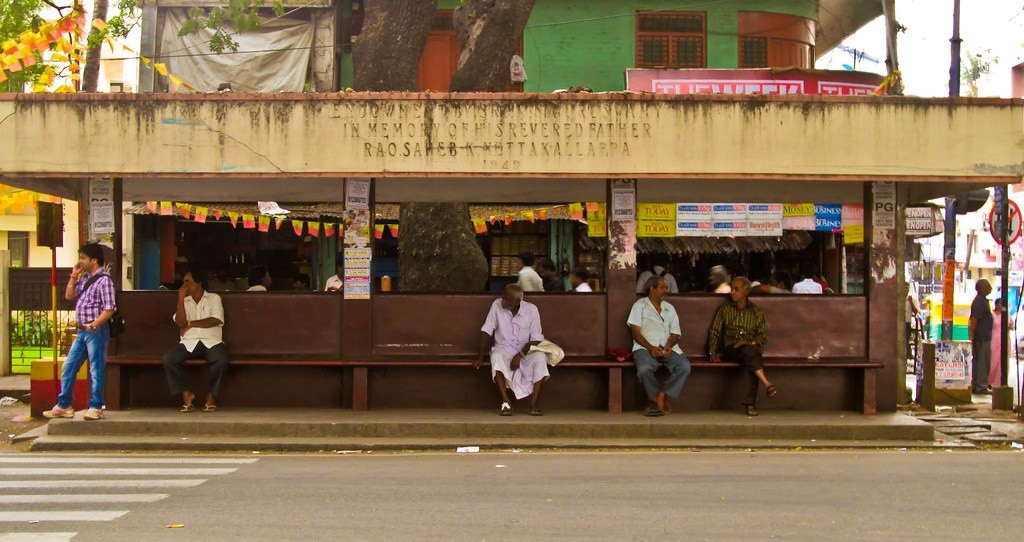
[528, 341, 564, 367]
[108, 311, 124, 338]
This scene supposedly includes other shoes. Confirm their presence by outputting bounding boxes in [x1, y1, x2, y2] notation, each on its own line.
[203, 404, 216, 411]
[529, 407, 544, 415]
[501, 402, 512, 415]
[84, 407, 104, 419]
[746, 405, 758, 416]
[43, 405, 74, 418]
[766, 385, 781, 400]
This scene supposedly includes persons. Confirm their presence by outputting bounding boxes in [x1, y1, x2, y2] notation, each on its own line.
[748, 266, 826, 293]
[708, 277, 783, 416]
[905, 282, 918, 360]
[472, 284, 550, 416]
[626, 275, 690, 415]
[162, 266, 228, 412]
[969, 279, 1014, 394]
[515, 252, 592, 292]
[709, 265, 731, 293]
[635, 262, 679, 293]
[246, 265, 271, 292]
[324, 262, 344, 291]
[293, 273, 310, 288]
[42, 242, 118, 420]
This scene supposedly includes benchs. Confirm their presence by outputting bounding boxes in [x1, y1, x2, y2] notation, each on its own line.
[105, 353, 888, 417]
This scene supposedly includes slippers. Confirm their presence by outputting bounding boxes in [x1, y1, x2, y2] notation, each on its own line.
[642, 410, 664, 416]
[180, 404, 196, 412]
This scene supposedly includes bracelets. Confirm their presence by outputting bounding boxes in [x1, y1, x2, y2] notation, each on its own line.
[752, 342, 755, 345]
[709, 353, 715, 355]
[69, 274, 77, 278]
[520, 350, 524, 357]
[90, 325, 96, 330]
[188, 320, 190, 325]
